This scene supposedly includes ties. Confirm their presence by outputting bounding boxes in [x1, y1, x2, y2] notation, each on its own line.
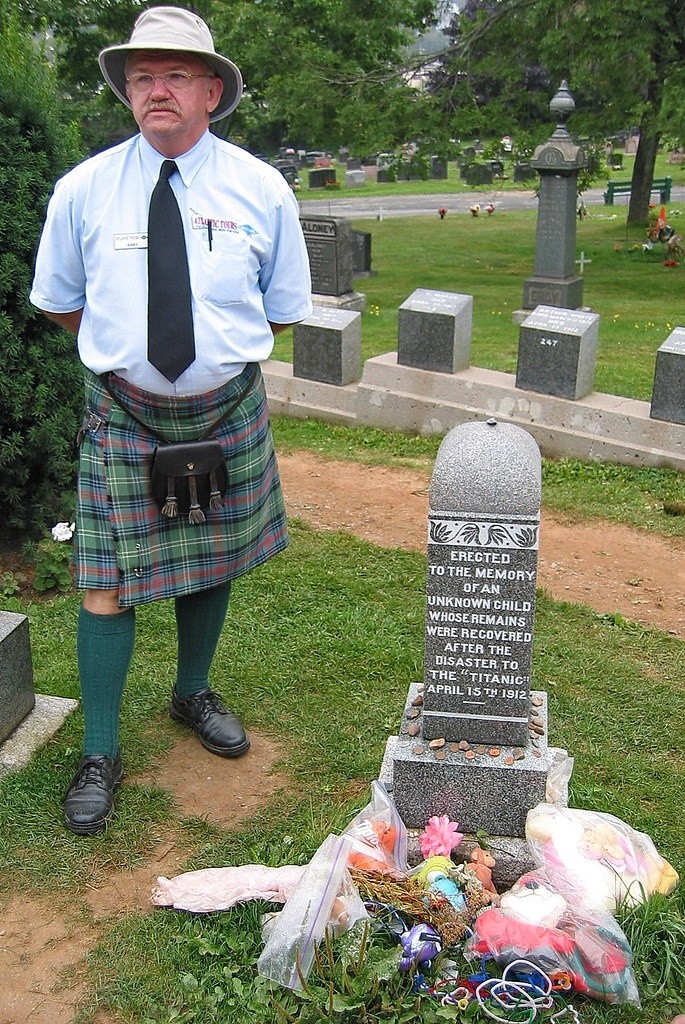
[147, 160, 196, 384]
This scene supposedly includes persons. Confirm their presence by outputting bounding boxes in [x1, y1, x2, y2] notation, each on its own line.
[30, 6, 314, 833]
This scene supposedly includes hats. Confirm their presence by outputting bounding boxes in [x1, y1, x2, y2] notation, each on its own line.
[98, 6, 244, 124]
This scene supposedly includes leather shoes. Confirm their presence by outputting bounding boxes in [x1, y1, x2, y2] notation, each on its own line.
[61, 749, 123, 834]
[169, 683, 250, 757]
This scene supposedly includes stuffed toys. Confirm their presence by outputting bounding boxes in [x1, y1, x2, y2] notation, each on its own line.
[331, 811, 679, 998]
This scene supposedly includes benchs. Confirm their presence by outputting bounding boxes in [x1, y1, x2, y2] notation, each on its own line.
[602, 174, 674, 205]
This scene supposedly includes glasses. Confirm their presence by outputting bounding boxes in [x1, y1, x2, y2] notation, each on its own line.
[126, 70, 214, 92]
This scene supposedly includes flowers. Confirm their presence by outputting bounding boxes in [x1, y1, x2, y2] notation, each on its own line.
[418, 816, 515, 860]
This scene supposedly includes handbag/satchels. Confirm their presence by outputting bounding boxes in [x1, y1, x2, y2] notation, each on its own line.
[151, 437, 228, 524]
